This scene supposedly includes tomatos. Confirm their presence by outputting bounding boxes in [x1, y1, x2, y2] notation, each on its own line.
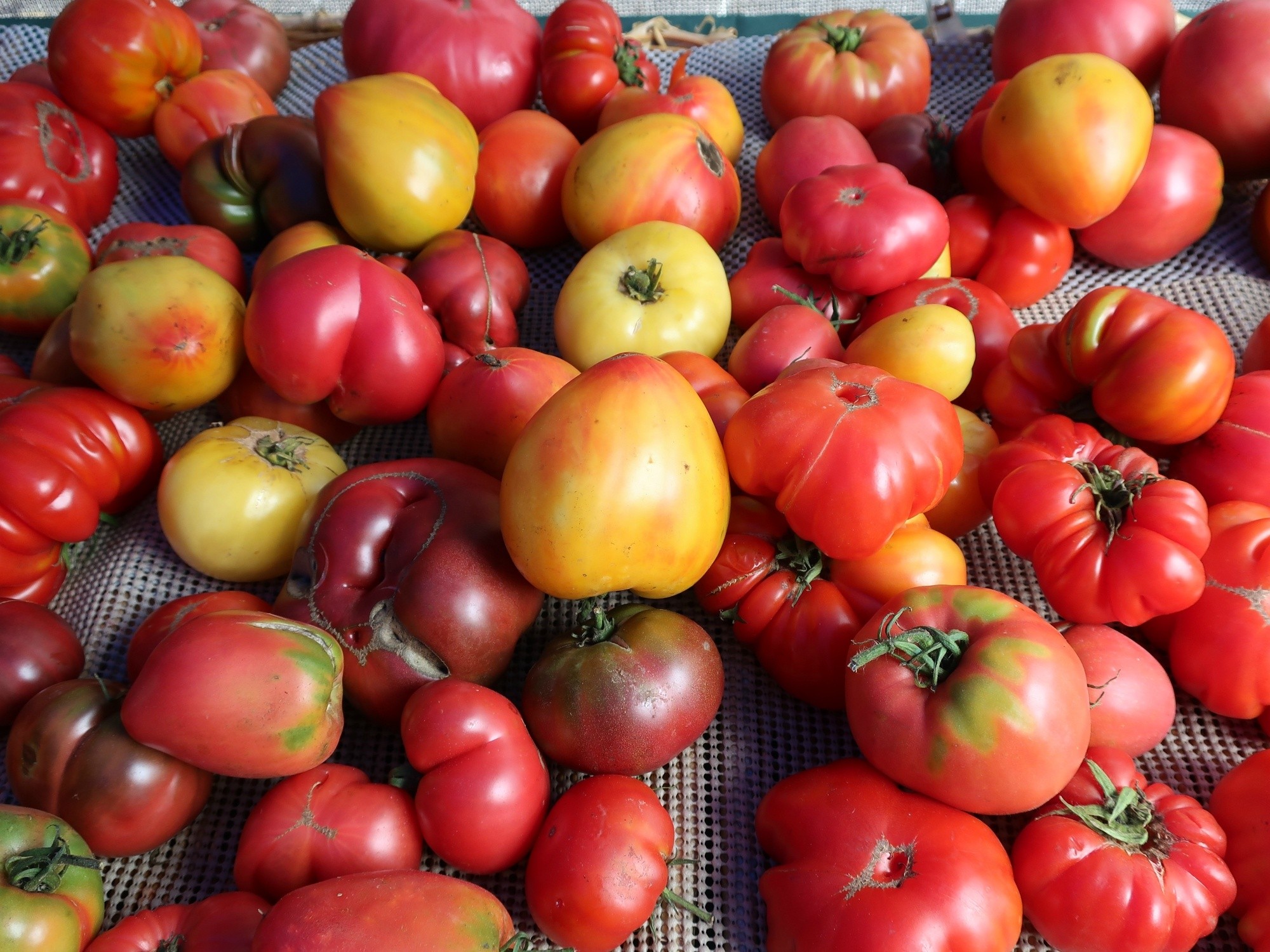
[0, 0, 1270, 952]
